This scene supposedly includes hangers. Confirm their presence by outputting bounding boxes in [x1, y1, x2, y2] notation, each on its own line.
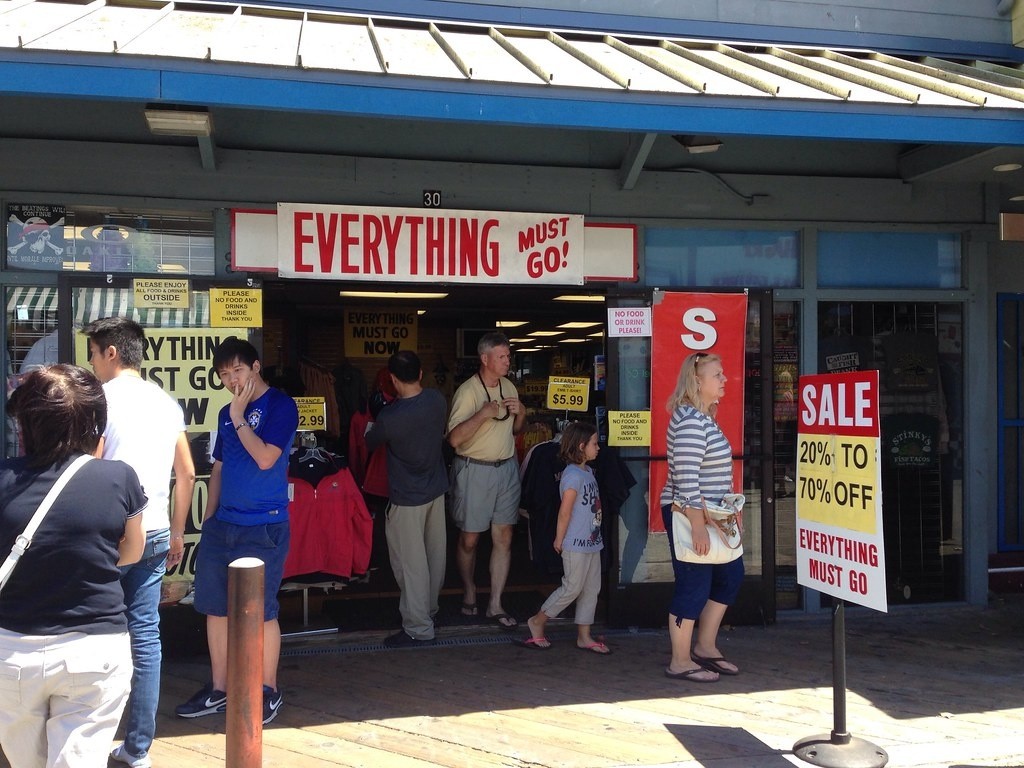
[550, 419, 576, 445]
[289, 431, 340, 465]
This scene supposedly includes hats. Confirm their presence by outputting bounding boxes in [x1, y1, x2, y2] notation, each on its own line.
[379, 368, 398, 400]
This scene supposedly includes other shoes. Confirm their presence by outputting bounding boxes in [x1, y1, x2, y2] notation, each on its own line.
[383, 630, 436, 647]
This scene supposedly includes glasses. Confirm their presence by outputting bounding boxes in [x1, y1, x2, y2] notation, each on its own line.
[694, 352, 708, 367]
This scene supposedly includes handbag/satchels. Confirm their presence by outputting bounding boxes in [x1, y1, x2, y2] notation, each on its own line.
[671, 492, 747, 564]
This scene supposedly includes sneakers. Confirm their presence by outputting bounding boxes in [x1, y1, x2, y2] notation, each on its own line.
[263, 684, 286, 725]
[111, 744, 152, 768]
[176, 682, 227, 719]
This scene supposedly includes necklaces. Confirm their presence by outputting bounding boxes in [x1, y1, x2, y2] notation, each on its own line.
[476, 369, 510, 421]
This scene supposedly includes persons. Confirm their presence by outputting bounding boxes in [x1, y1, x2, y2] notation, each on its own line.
[364, 350, 450, 648]
[773, 371, 793, 420]
[6, 362, 45, 459]
[442, 332, 527, 631]
[511, 422, 612, 656]
[81, 318, 196, 768]
[175, 340, 299, 725]
[0, 361, 149, 768]
[658, 351, 745, 683]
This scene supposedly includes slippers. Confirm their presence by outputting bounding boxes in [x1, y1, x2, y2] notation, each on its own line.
[487, 613, 517, 630]
[458, 602, 479, 619]
[576, 640, 612, 655]
[690, 650, 739, 674]
[510, 634, 547, 648]
[664, 664, 720, 682]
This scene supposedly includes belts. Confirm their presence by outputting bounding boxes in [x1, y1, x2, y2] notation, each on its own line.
[457, 452, 515, 467]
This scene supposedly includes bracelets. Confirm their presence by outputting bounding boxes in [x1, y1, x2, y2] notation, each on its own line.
[235, 423, 249, 432]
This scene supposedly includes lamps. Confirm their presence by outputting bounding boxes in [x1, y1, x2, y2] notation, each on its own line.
[340, 290, 605, 353]
[145, 103, 212, 135]
[673, 132, 724, 152]
